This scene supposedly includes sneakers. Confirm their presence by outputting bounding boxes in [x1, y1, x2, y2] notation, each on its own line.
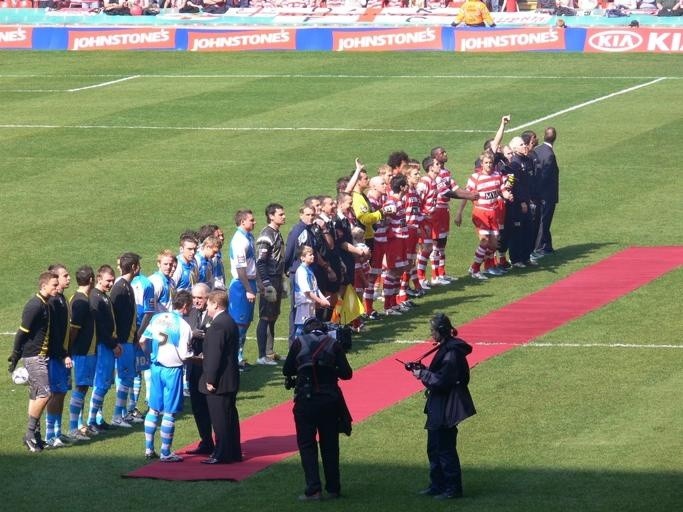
[85, 423, 106, 435]
[419, 487, 440, 495]
[266, 352, 280, 360]
[299, 489, 321, 501]
[160, 451, 184, 462]
[257, 356, 278, 365]
[466, 248, 557, 279]
[18, 408, 146, 450]
[432, 490, 464, 500]
[145, 449, 156, 459]
[363, 300, 416, 315]
[403, 273, 460, 294]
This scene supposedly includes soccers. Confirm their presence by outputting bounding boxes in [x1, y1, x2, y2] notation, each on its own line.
[12, 367, 30, 385]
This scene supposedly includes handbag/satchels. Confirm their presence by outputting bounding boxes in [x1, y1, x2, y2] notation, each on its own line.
[289, 321, 352, 350]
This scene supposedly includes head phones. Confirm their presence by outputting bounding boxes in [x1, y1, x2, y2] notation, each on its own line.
[302, 318, 327, 333]
[437, 312, 448, 337]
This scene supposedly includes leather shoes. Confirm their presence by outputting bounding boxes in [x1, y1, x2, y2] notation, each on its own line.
[209, 449, 217, 458]
[185, 445, 208, 454]
[201, 456, 230, 464]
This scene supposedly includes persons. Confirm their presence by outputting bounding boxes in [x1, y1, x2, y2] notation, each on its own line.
[412, 312, 477, 500]
[183, 283, 213, 454]
[0, 0, 683, 30]
[138, 289, 197, 461]
[198, 288, 245, 464]
[282, 316, 353, 499]
[228, 146, 480, 371]
[6, 225, 227, 454]
[448, 0, 497, 28]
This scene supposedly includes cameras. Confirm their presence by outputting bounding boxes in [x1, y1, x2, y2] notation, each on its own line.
[405, 361, 427, 372]
[284, 376, 296, 389]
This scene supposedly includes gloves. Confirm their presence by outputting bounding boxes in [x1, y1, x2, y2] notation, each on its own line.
[8, 353, 19, 373]
[263, 286, 277, 302]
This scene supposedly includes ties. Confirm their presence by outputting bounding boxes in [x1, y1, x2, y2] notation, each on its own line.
[196, 311, 202, 329]
